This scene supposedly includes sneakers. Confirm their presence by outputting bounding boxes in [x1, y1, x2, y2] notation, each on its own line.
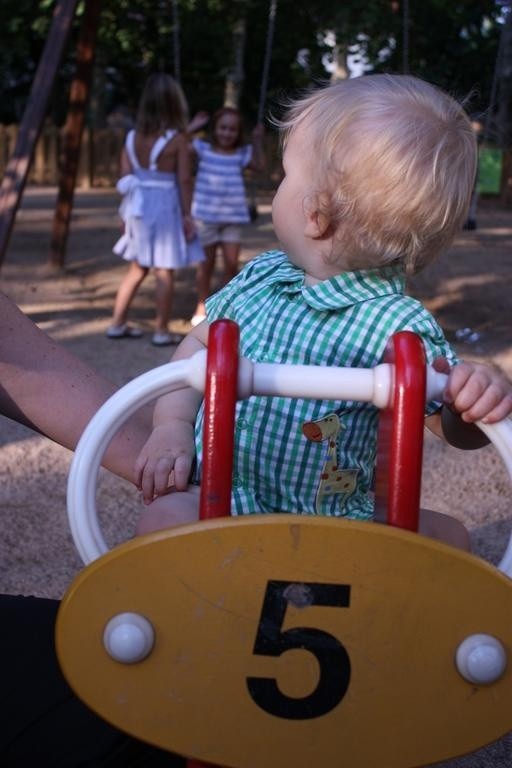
[191, 315, 207, 325]
[151, 335, 184, 346]
[107, 324, 143, 337]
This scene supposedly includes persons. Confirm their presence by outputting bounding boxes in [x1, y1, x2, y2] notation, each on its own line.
[0, 291, 187, 768]
[105, 72, 207, 344]
[132, 75, 512, 555]
[185, 107, 266, 328]
